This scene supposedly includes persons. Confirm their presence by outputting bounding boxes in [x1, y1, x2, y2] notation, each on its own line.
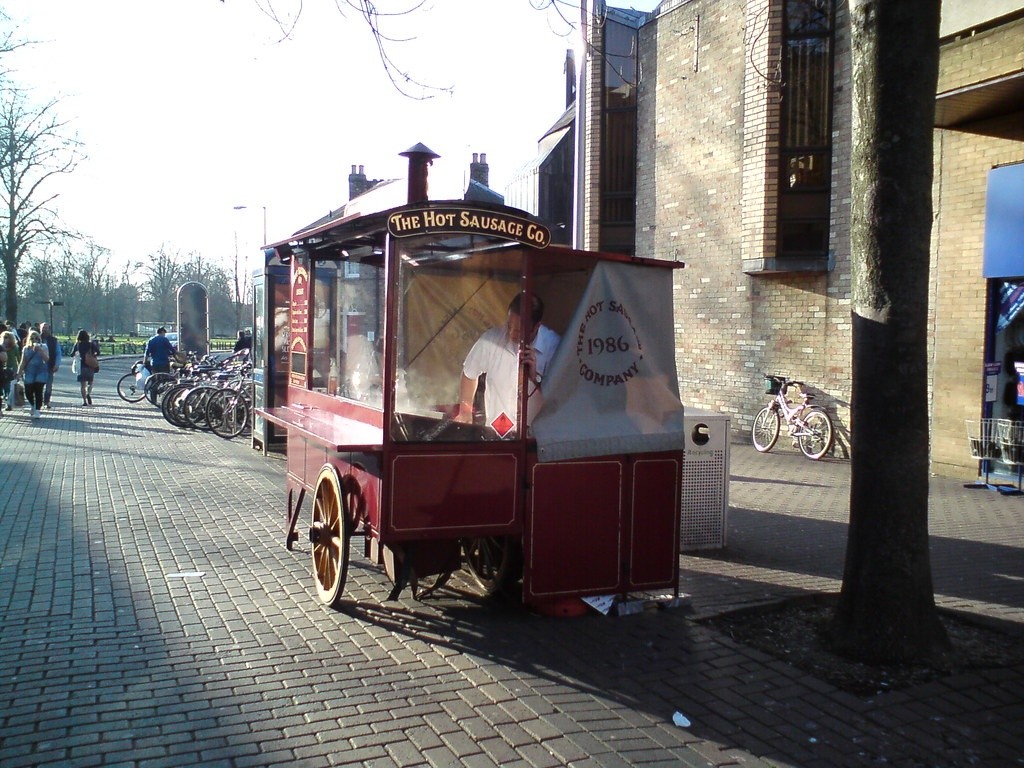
[0, 317, 42, 420]
[232, 330, 252, 354]
[69, 329, 101, 406]
[454, 290, 563, 433]
[17, 330, 50, 420]
[37, 321, 62, 409]
[144, 327, 178, 390]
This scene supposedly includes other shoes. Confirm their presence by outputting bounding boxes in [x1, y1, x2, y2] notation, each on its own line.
[30, 406, 34, 415]
[44, 403, 51, 408]
[35, 410, 39, 418]
[83, 403, 87, 406]
[87, 394, 91, 404]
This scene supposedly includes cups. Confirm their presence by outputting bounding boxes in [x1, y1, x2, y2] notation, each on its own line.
[34, 343, 38, 351]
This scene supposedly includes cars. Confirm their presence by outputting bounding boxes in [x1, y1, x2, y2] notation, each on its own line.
[165, 332, 177, 348]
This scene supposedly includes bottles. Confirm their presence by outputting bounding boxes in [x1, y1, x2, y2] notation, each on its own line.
[2, 352, 7, 369]
[328, 363, 339, 395]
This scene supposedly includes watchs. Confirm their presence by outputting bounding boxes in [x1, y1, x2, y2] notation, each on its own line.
[532, 372, 541, 386]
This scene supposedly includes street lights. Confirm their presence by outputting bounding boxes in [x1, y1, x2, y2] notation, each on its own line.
[35, 298, 65, 334]
[233, 205, 267, 246]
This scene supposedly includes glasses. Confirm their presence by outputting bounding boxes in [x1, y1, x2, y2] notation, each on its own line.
[31, 338, 38, 340]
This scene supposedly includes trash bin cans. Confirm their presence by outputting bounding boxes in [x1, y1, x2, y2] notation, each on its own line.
[680, 406, 731, 551]
[130, 331, 134, 337]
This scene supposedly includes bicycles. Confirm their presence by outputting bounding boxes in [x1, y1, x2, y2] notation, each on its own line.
[116, 342, 252, 439]
[750, 374, 835, 461]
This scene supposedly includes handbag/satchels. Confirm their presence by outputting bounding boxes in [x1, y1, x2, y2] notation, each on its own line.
[71, 342, 81, 375]
[7, 379, 24, 409]
[135, 366, 152, 390]
[85, 343, 98, 368]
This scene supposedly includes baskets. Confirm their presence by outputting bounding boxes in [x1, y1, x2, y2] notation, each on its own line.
[764, 375, 787, 395]
